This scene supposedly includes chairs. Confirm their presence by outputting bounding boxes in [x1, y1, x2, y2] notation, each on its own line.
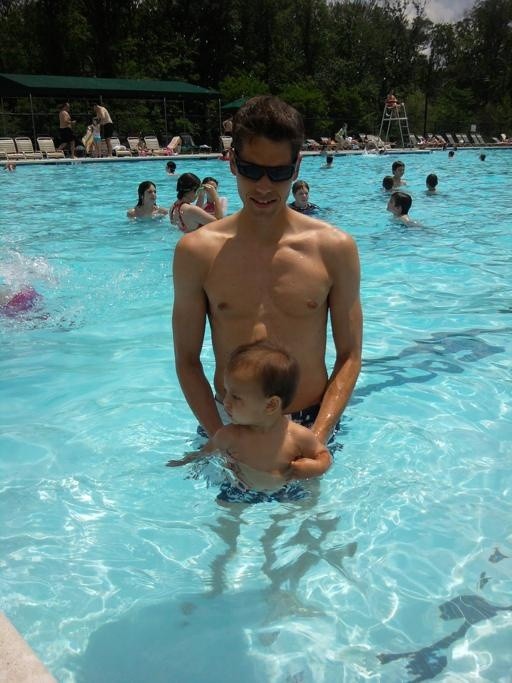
[15, 136, 43, 160]
[126, 136, 147, 156]
[36, 136, 66, 159]
[220, 134, 235, 152]
[299, 133, 512, 148]
[181, 133, 212, 154]
[141, 135, 163, 155]
[0, 135, 24, 163]
[107, 136, 132, 156]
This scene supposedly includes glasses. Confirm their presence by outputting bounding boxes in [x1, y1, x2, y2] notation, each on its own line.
[235, 150, 296, 182]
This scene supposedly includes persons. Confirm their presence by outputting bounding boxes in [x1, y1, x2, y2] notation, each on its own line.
[127, 161, 227, 231]
[171, 92, 363, 485]
[300, 89, 511, 169]
[1, 611, 62, 681]
[382, 161, 438, 222]
[162, 345, 335, 502]
[53, 102, 235, 160]
[0, 283, 49, 330]
[286, 180, 321, 212]
[5, 162, 17, 173]
